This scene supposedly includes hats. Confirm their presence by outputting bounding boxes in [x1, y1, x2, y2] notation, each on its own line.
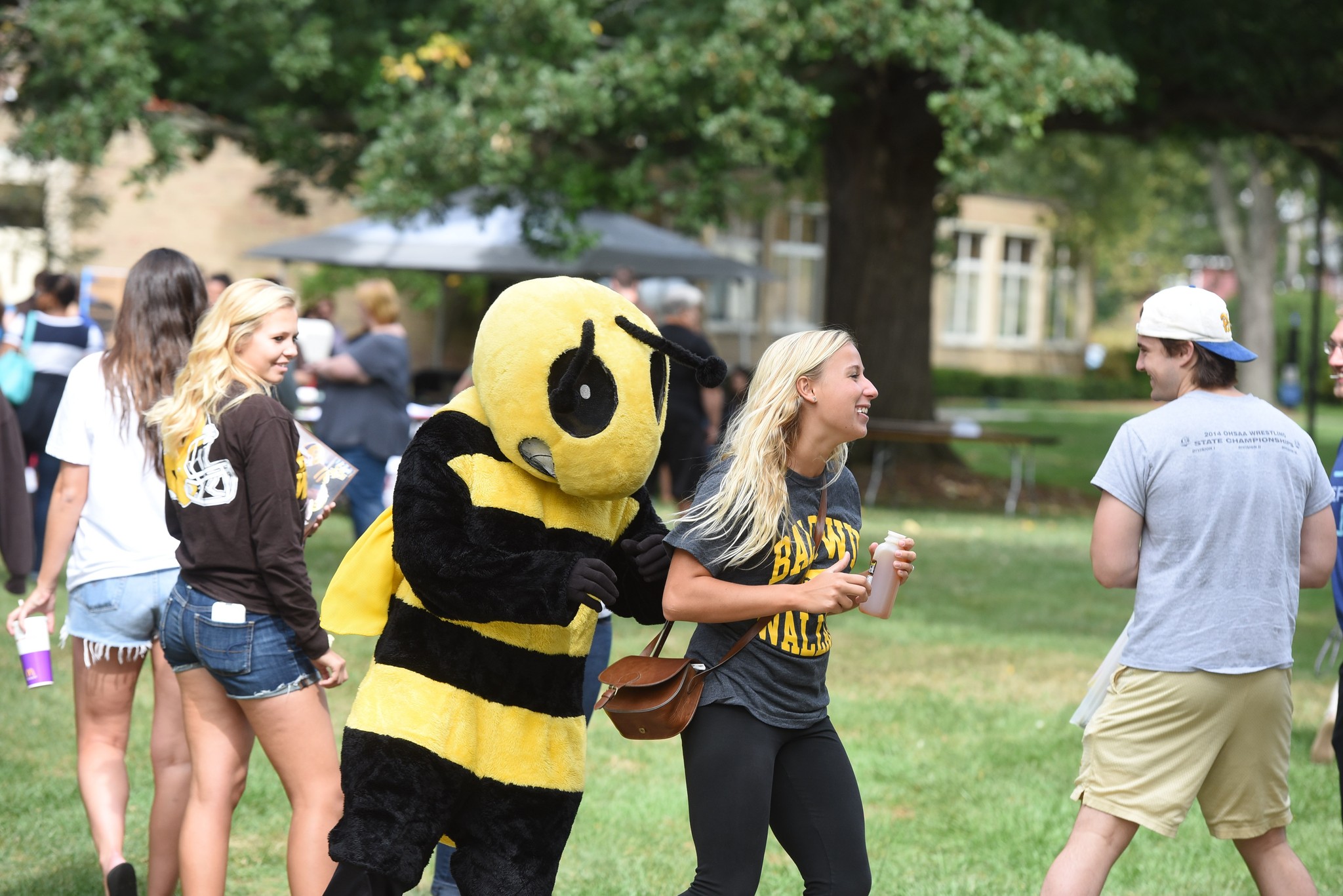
[1136, 282, 1258, 363]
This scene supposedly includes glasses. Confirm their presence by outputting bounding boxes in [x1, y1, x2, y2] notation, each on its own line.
[1320, 341, 1343, 356]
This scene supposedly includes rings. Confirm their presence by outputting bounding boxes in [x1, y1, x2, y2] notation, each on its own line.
[17, 598, 25, 609]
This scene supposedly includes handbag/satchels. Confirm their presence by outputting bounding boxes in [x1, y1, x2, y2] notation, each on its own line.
[592, 655, 704, 741]
[0, 310, 38, 405]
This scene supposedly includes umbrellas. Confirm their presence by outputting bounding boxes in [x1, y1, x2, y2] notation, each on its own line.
[238, 172, 793, 289]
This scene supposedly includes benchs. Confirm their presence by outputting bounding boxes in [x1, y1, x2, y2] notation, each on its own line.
[849, 411, 1065, 517]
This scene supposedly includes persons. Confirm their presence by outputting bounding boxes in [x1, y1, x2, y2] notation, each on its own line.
[7, 249, 210, 896]
[0, 220, 481, 591]
[632, 271, 789, 524]
[1317, 300, 1343, 838]
[149, 274, 351, 896]
[658, 322, 917, 896]
[1036, 281, 1325, 896]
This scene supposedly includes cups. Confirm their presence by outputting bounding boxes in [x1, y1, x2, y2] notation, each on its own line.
[11, 615, 54, 689]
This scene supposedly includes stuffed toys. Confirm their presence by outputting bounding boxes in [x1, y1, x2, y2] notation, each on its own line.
[319, 279, 680, 896]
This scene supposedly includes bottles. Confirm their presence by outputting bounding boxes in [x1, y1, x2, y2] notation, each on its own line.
[859, 530, 906, 620]
